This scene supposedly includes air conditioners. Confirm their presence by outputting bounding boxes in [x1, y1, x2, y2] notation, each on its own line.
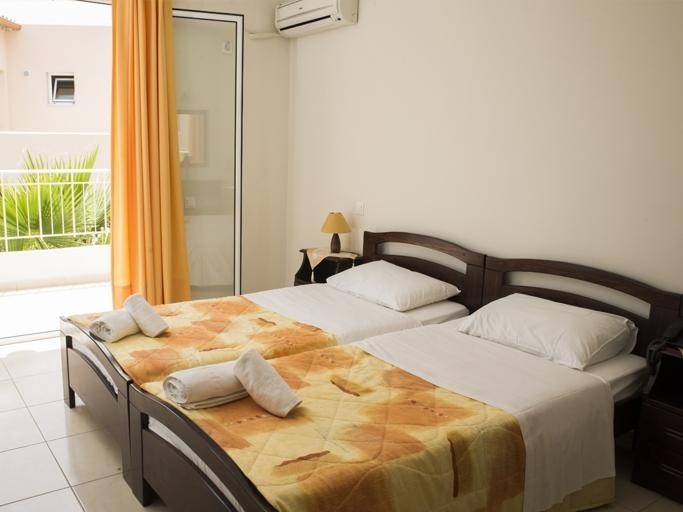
[270, 0, 360, 39]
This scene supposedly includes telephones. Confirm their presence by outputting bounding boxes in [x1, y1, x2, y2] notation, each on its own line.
[663, 317, 683, 350]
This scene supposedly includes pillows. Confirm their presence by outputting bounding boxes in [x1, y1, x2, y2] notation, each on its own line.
[458, 292, 637, 372]
[326, 260, 460, 313]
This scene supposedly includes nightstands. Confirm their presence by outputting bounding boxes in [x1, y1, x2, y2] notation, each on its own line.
[631, 342, 682, 504]
[291, 247, 357, 286]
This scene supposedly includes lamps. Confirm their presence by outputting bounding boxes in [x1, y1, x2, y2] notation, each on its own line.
[319, 212, 350, 252]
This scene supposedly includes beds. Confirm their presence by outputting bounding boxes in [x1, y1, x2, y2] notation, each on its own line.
[59, 230, 485, 493]
[127, 259, 660, 512]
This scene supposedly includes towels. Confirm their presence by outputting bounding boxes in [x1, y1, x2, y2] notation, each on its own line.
[120, 290, 169, 339]
[233, 348, 302, 419]
[161, 361, 248, 412]
[88, 306, 138, 345]
